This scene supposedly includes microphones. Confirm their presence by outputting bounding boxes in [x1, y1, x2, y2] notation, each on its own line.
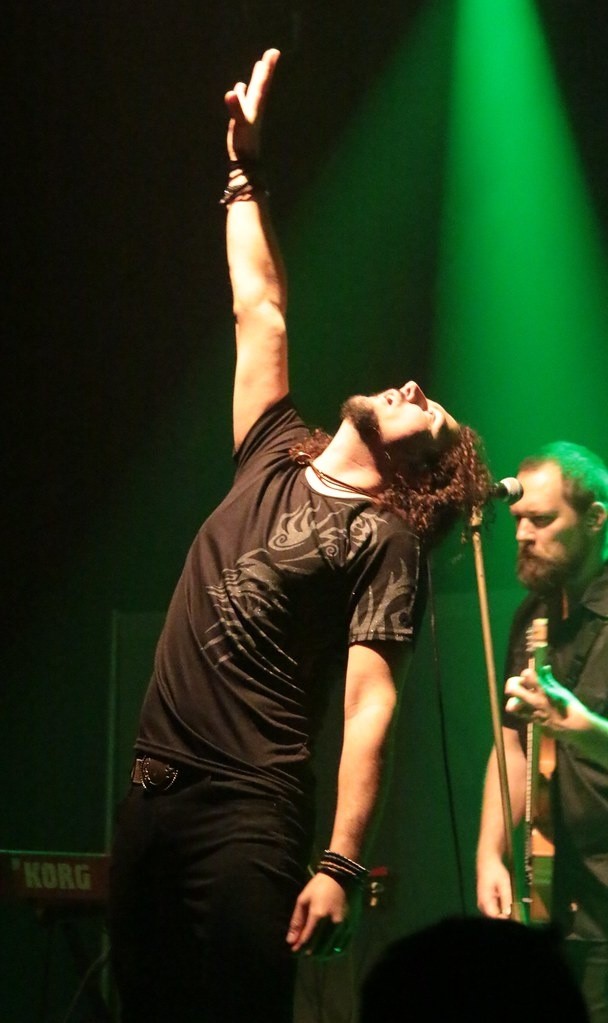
[491, 476, 524, 505]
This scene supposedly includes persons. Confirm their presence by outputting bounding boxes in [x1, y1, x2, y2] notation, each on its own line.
[474, 440, 608, 1023]
[359, 916, 592, 1023]
[111, 48, 495, 1023]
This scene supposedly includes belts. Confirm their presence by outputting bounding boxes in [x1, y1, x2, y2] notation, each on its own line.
[131, 755, 194, 793]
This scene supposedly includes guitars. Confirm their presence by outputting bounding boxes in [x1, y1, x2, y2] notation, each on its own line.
[505, 617, 557, 929]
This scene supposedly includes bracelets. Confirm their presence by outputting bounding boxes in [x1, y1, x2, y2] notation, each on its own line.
[219, 161, 262, 205]
[320, 849, 367, 889]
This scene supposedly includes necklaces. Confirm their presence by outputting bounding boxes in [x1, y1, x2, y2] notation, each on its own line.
[295, 452, 374, 496]
